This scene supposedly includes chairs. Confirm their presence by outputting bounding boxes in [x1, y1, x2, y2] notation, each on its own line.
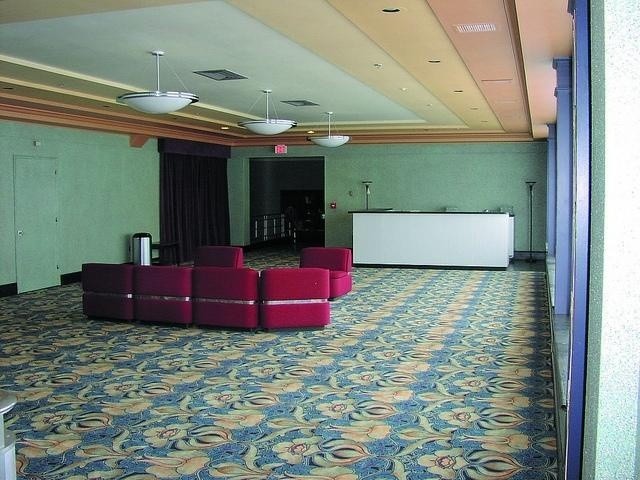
[82, 244, 353, 333]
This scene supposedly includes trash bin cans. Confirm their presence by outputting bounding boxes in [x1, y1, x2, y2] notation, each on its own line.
[0, 389, 18, 480]
[131, 232, 152, 266]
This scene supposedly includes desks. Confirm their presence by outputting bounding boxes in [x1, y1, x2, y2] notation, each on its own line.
[150, 241, 183, 265]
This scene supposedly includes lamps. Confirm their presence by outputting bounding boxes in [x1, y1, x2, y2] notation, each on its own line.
[362, 181, 373, 210]
[236, 89, 295, 136]
[306, 112, 352, 149]
[525, 182, 536, 264]
[116, 50, 199, 114]
